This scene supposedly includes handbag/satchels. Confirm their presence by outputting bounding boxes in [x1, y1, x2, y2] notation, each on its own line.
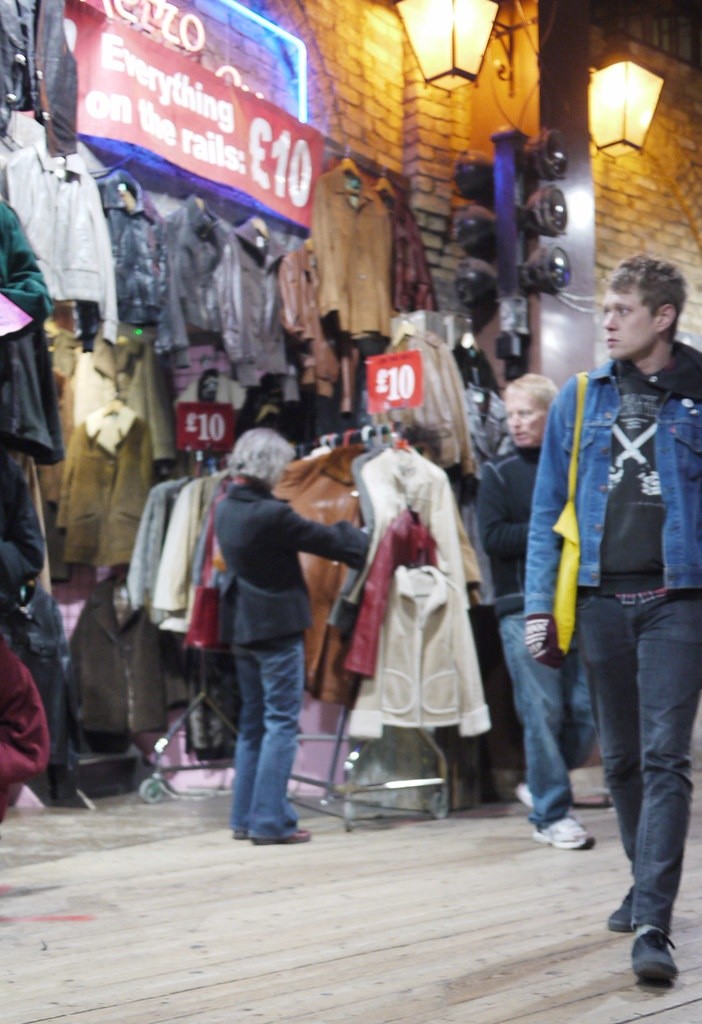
[185, 588, 220, 647]
[552, 502, 581, 653]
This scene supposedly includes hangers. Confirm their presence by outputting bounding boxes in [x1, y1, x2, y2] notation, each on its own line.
[100, 392, 127, 417]
[57, 145, 398, 255]
[184, 395, 416, 484]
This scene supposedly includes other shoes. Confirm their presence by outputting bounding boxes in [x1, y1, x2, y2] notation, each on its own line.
[233, 829, 246, 839]
[283, 830, 308, 842]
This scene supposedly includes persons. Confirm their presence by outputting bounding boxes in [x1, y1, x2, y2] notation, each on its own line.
[472, 372, 597, 852]
[522, 254, 702, 984]
[214, 428, 370, 844]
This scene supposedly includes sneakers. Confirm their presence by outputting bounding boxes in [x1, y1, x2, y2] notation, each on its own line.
[532, 817, 594, 849]
[631, 923, 680, 983]
[513, 784, 532, 810]
[608, 886, 631, 931]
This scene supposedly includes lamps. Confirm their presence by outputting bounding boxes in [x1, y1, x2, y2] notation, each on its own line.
[394, 0, 500, 95]
[587, 48, 663, 157]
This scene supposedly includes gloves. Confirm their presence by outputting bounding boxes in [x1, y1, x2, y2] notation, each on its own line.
[524, 613, 561, 665]
